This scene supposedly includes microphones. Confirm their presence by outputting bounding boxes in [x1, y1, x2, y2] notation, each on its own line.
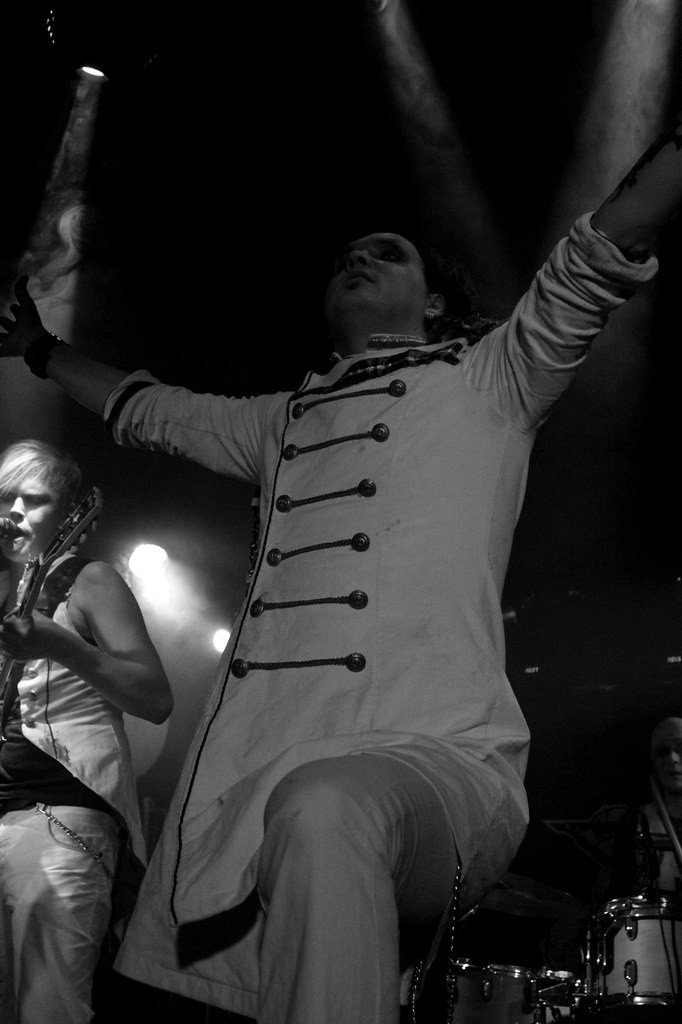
[639, 815, 662, 881]
[0, 517, 20, 542]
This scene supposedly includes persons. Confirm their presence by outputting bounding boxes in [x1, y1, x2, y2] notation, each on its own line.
[638, 715, 682, 895]
[0, 439, 173, 1023]
[0, 117, 682, 1024]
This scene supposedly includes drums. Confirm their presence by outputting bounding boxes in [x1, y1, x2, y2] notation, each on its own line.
[449, 960, 575, 1024]
[585, 889, 679, 1008]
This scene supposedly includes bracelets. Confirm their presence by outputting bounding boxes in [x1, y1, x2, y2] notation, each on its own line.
[20, 334, 73, 382]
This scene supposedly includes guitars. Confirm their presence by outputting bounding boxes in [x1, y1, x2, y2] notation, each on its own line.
[1, 484, 105, 731]
